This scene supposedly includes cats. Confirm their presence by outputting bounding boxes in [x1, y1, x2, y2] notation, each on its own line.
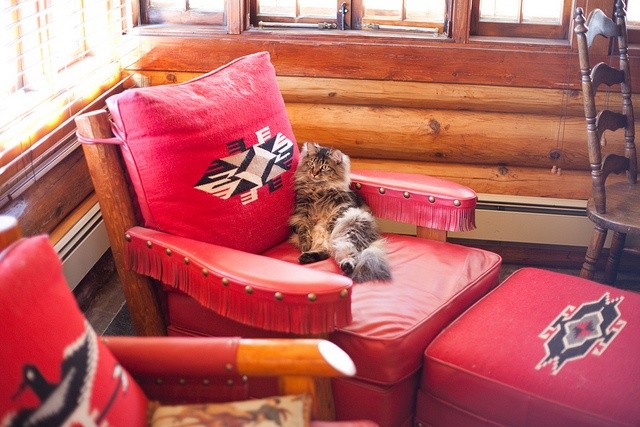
[288, 141, 393, 283]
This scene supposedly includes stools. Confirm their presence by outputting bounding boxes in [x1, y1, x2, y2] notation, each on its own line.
[416, 267, 639, 425]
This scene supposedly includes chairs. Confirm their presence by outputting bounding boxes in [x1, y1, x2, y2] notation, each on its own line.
[73, 74, 500, 427]
[0, 216, 382, 427]
[572, 0, 639, 280]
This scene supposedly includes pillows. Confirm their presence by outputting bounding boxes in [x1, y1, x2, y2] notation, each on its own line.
[107, 52, 301, 254]
[0, 234, 149, 427]
